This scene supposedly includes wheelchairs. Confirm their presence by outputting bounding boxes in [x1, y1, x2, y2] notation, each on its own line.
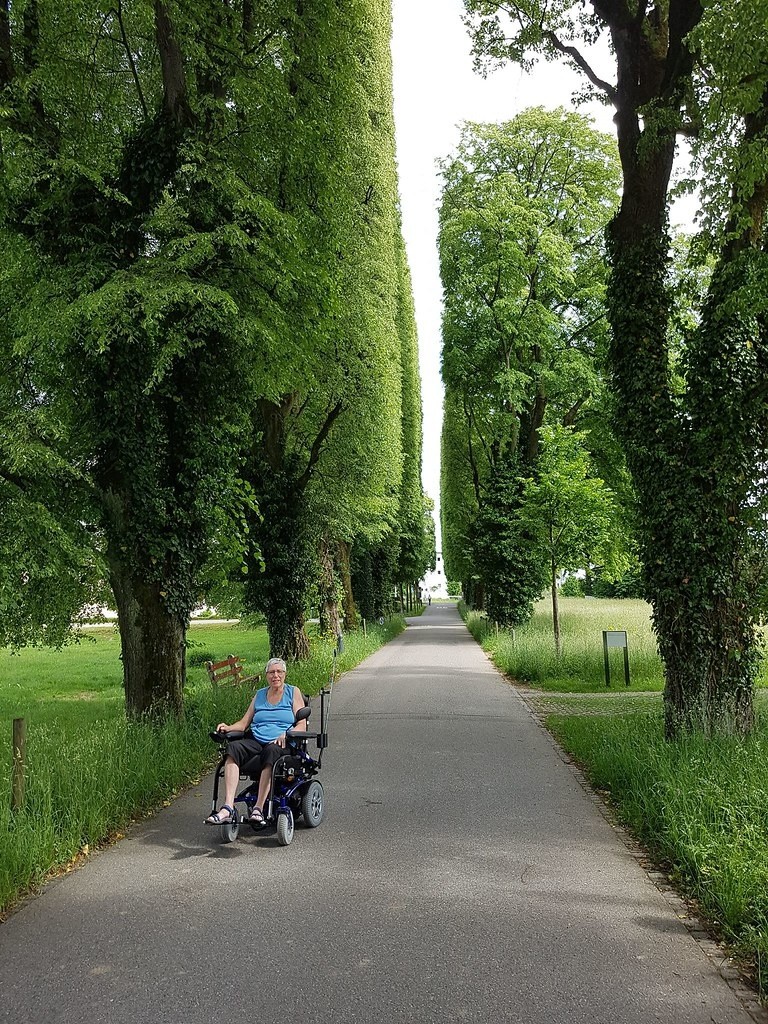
[203, 687, 332, 846]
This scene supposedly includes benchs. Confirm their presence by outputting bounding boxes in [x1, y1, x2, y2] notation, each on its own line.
[205, 653, 263, 690]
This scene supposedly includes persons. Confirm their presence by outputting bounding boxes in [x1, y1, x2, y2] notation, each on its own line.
[428, 594, 431, 605]
[203, 658, 305, 824]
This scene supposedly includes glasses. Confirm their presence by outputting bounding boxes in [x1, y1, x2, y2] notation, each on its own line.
[266, 669, 285, 674]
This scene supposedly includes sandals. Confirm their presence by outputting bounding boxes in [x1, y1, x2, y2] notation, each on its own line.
[204, 805, 234, 824]
[249, 807, 264, 822]
